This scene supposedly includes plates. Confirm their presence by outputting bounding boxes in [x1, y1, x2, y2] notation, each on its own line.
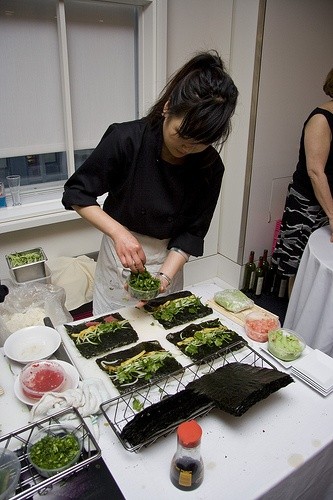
[259, 340, 313, 368]
[290, 349, 333, 397]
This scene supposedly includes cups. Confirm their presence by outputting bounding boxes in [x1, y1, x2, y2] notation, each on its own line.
[0, 181, 7, 207]
[6, 175, 21, 205]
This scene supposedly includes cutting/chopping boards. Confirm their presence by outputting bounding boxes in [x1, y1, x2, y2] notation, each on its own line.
[207, 294, 281, 327]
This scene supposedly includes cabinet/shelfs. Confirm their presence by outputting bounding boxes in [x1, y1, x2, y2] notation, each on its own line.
[217, 0, 333, 294]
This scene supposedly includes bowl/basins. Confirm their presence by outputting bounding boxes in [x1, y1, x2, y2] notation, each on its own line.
[14, 360, 80, 406]
[0, 447, 21, 500]
[4, 325, 62, 364]
[5, 247, 52, 289]
[127, 275, 160, 300]
[26, 425, 84, 478]
[19, 359, 65, 401]
[246, 311, 281, 342]
[267, 329, 306, 361]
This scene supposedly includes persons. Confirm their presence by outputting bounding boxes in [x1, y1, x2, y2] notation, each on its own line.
[61, 50, 239, 316]
[269, 68, 333, 296]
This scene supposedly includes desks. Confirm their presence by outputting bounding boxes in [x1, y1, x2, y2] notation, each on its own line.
[276, 224, 333, 356]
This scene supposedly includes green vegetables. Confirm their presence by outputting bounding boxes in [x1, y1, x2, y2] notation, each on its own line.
[97, 321, 123, 332]
[135, 294, 202, 326]
[268, 330, 303, 362]
[29, 432, 80, 470]
[176, 318, 234, 355]
[105, 348, 171, 412]
[129, 266, 160, 300]
[215, 290, 251, 312]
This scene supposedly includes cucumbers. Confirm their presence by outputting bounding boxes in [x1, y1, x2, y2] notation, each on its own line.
[7, 252, 42, 268]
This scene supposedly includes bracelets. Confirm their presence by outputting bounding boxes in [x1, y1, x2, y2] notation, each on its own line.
[170, 248, 188, 262]
[159, 272, 171, 287]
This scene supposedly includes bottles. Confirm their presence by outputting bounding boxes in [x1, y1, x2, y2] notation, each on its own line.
[243, 251, 257, 293]
[253, 249, 289, 299]
[169, 420, 203, 491]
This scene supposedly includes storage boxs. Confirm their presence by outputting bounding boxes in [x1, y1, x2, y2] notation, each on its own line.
[5, 247, 48, 281]
